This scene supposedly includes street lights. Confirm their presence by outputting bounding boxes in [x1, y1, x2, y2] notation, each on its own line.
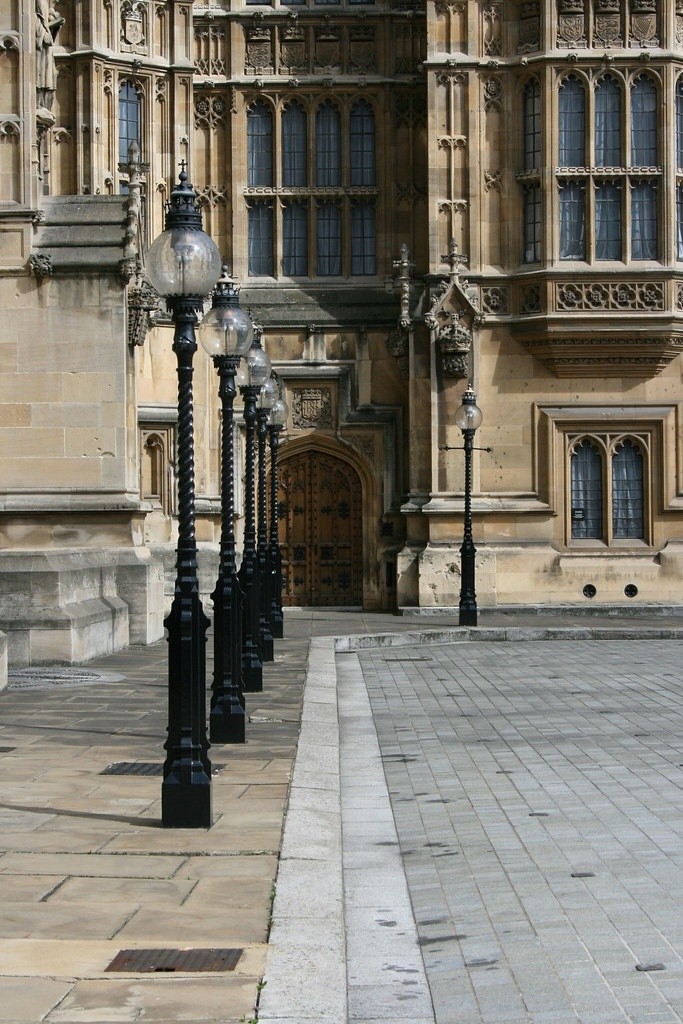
[254, 375, 281, 665]
[196, 270, 255, 748]
[140, 161, 223, 830]
[231, 322, 273, 699]
[265, 397, 289, 641]
[453, 383, 484, 627]
